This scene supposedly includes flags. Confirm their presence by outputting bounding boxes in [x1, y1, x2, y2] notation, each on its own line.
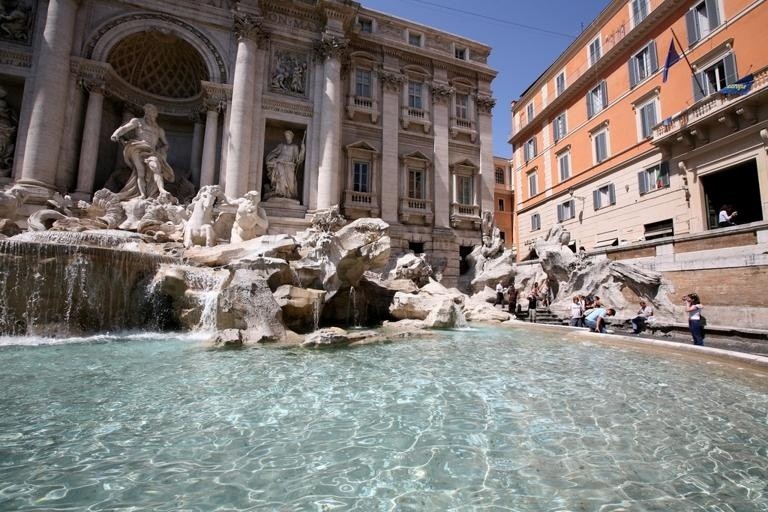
[663, 37, 682, 83]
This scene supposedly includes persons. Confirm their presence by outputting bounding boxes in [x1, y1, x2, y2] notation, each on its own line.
[630, 302, 654, 334]
[291, 66, 303, 90]
[527, 280, 550, 323]
[683, 293, 704, 346]
[108, 103, 175, 197]
[718, 205, 739, 226]
[272, 72, 288, 89]
[570, 294, 616, 333]
[264, 130, 305, 198]
[508, 284, 518, 313]
[493, 280, 505, 308]
